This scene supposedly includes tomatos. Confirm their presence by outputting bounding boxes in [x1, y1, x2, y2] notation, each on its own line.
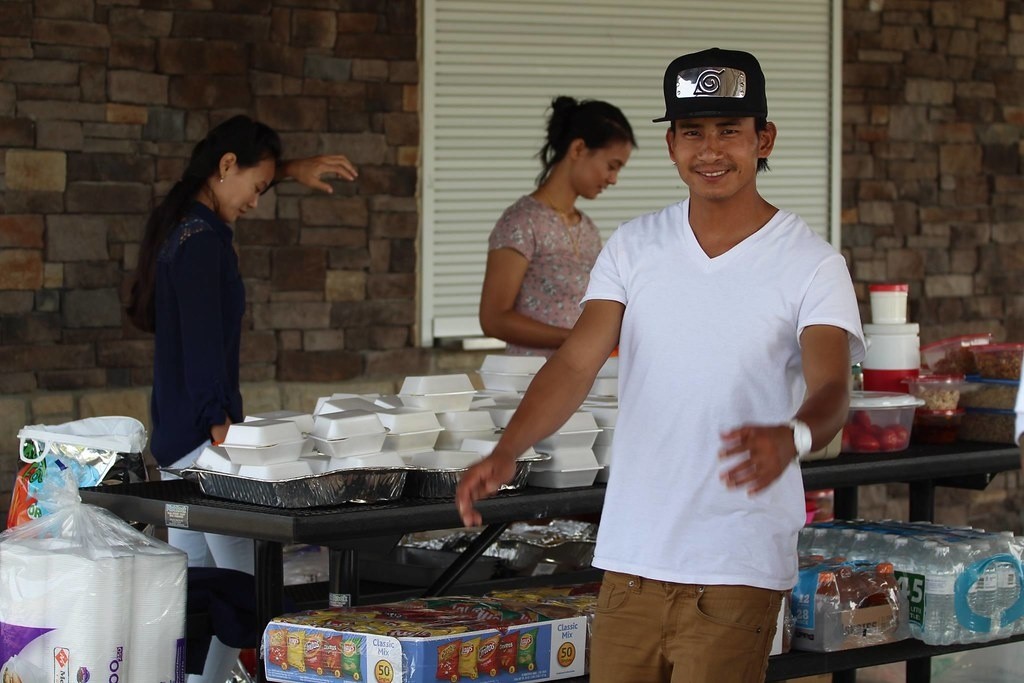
[843, 410, 908, 451]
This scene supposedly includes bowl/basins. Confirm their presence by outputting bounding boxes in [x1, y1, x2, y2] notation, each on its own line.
[840, 333, 1024, 452]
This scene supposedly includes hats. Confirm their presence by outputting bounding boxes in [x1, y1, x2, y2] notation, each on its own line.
[652, 47, 768, 123]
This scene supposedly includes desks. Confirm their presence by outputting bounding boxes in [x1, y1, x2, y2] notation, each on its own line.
[77, 439, 1022, 683]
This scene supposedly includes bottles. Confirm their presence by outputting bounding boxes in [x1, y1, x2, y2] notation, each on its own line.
[783, 518, 1024, 652]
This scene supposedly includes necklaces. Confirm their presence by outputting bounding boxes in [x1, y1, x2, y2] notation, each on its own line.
[542, 190, 583, 257]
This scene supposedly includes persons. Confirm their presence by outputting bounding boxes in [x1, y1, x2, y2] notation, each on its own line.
[454, 47, 869, 683]
[136, 114, 360, 683]
[1013, 357, 1024, 474]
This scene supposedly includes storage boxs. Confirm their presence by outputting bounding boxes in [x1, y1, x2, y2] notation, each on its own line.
[259, 551, 914, 683]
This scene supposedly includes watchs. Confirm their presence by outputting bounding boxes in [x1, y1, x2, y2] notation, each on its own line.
[786, 418, 813, 463]
